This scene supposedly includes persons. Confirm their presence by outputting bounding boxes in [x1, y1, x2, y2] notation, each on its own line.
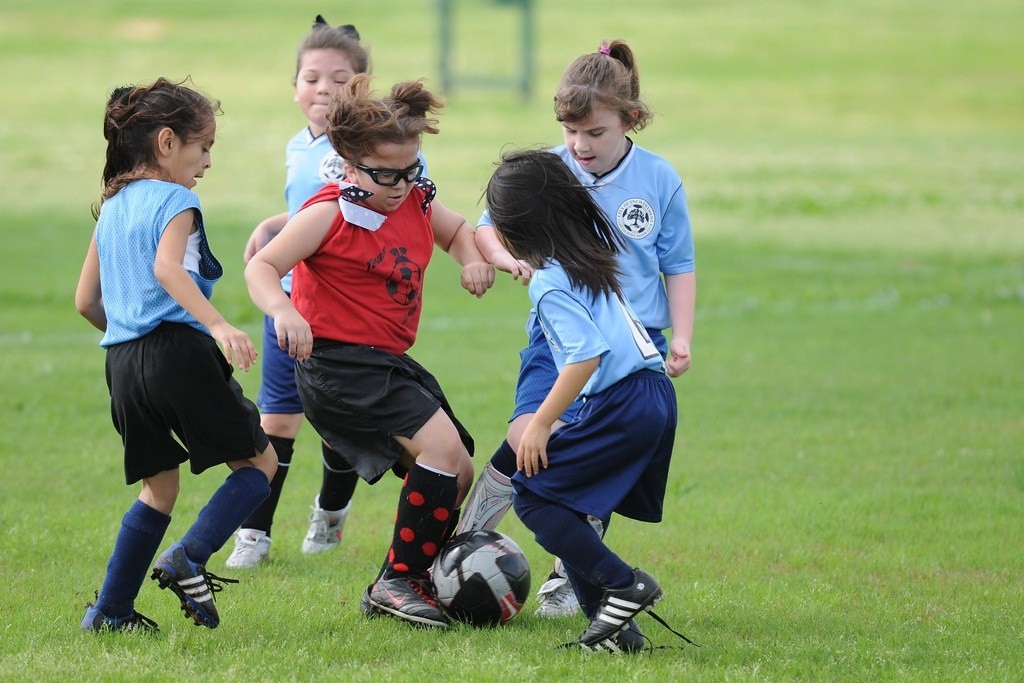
[457, 39, 696, 655]
[75, 78, 278, 633]
[228, 17, 494, 628]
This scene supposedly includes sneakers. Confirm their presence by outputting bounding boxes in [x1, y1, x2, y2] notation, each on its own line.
[149, 542, 239, 629]
[225, 528, 273, 568]
[301, 493, 352, 555]
[360, 568, 451, 631]
[80, 605, 160, 637]
[554, 618, 653, 656]
[576, 567, 701, 648]
[533, 571, 580, 619]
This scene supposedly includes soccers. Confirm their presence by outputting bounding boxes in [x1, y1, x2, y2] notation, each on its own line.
[435, 529, 531, 623]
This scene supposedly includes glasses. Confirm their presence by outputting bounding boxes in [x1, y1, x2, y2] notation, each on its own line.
[345, 158, 424, 186]
